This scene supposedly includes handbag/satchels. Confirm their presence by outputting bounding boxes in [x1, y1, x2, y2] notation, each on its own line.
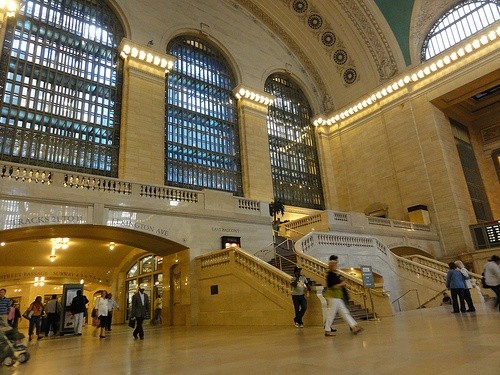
[22, 303, 34, 319]
[93, 317, 100, 326]
[128, 316, 136, 328]
[91, 307, 96, 317]
[55, 313, 60, 321]
[83, 296, 87, 317]
[343, 287, 350, 301]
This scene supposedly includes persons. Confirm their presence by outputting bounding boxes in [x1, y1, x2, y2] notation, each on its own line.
[290, 267, 311, 328]
[483, 255, 500, 311]
[324, 255, 362, 336]
[93, 290, 119, 338]
[23, 294, 61, 341]
[132, 286, 150, 340]
[273, 218, 280, 236]
[442, 293, 451, 305]
[0, 289, 28, 350]
[70, 290, 89, 335]
[446, 261, 476, 313]
[154, 295, 162, 319]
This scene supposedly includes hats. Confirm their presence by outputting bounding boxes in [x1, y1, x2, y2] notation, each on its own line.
[330, 255, 338, 260]
[294, 266, 302, 273]
[52, 295, 57, 299]
[139, 284, 145, 290]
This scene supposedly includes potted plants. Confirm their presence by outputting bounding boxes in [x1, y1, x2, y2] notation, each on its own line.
[269, 197, 285, 235]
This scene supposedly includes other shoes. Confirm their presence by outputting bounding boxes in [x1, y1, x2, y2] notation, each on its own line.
[466, 309, 475, 312]
[140, 336, 144, 339]
[295, 322, 298, 327]
[74, 333, 82, 336]
[331, 328, 336, 332]
[352, 326, 362, 334]
[325, 332, 336, 336]
[100, 335, 106, 338]
[300, 324, 304, 327]
[37, 336, 43, 341]
[133, 333, 138, 338]
[105, 326, 111, 331]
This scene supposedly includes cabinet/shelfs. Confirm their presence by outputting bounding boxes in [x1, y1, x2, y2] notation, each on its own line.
[221, 235, 242, 250]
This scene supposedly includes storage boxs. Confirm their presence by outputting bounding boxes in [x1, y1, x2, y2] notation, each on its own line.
[406, 205, 432, 227]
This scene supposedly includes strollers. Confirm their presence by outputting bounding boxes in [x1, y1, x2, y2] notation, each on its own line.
[0, 316, 30, 366]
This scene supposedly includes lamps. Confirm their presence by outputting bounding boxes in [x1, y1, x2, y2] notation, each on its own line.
[312, 25, 500, 127]
[34, 276, 45, 287]
[109, 241, 115, 250]
[117, 38, 178, 74]
[349, 268, 356, 276]
[231, 81, 274, 106]
[48, 238, 69, 262]
[0, 0, 20, 21]
